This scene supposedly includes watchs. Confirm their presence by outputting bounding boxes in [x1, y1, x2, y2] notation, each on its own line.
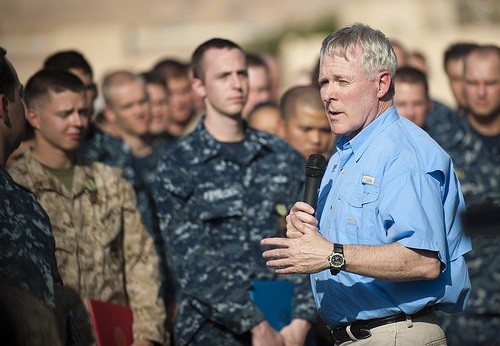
[328, 243, 346, 275]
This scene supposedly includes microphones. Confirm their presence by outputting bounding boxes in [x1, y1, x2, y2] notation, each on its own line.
[302, 153, 327, 235]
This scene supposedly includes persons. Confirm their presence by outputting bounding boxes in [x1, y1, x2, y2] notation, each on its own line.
[0, 38, 500, 346]
[260, 24, 472, 346]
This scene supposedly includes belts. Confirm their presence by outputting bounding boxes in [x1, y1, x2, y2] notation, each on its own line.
[329, 305, 434, 343]
